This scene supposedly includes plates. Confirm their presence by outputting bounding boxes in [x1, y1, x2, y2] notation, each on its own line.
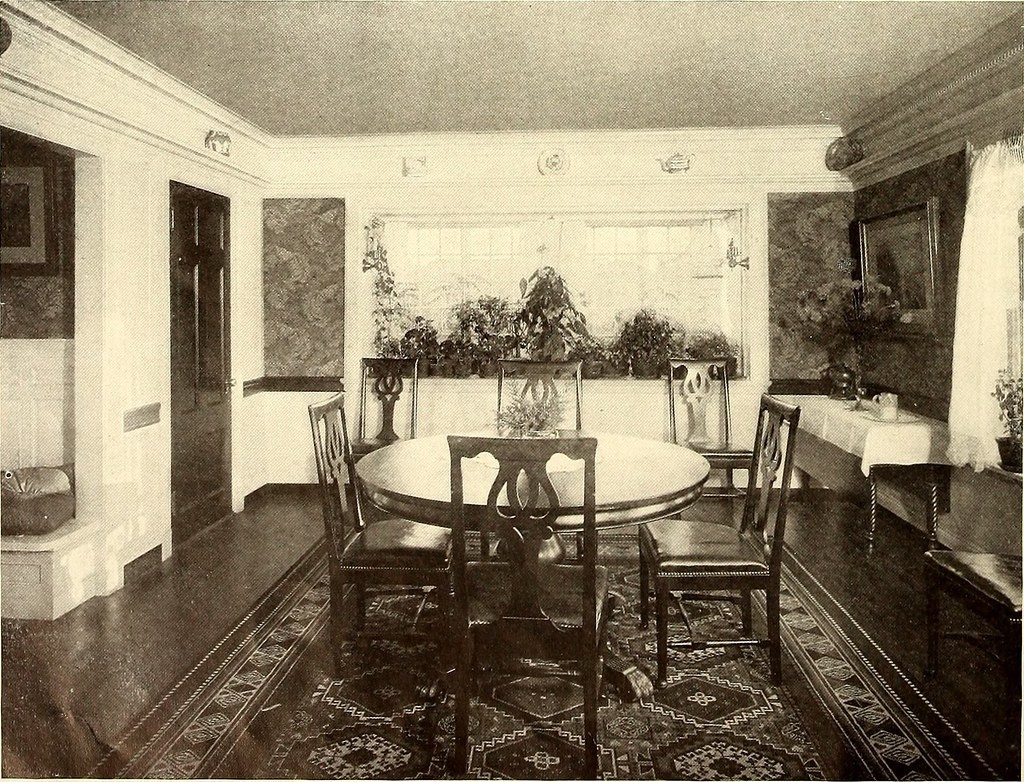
[861, 408, 922, 423]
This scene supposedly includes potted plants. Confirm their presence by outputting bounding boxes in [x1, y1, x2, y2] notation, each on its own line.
[360, 216, 741, 379]
[990, 368, 1024, 472]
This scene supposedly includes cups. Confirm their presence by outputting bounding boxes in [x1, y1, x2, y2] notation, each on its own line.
[873, 392, 899, 422]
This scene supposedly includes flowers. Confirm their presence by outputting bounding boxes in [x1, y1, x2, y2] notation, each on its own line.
[777, 272, 912, 346]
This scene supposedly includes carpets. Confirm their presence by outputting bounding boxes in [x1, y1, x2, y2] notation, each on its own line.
[84, 528, 1003, 782]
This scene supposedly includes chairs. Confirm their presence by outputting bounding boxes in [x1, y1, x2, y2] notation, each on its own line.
[667, 357, 755, 520]
[308, 390, 459, 679]
[446, 435, 609, 782]
[352, 355, 418, 465]
[497, 359, 581, 431]
[637, 393, 801, 689]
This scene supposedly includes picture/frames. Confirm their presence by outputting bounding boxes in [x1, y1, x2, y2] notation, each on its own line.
[849, 195, 947, 346]
[0, 151, 59, 277]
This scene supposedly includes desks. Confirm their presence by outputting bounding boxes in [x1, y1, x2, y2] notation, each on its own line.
[1, 520, 98, 621]
[770, 395, 959, 560]
[354, 429, 712, 621]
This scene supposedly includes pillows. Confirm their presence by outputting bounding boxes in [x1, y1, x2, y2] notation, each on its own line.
[1, 464, 75, 534]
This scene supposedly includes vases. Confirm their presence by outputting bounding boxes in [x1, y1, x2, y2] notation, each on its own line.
[832, 346, 863, 401]
